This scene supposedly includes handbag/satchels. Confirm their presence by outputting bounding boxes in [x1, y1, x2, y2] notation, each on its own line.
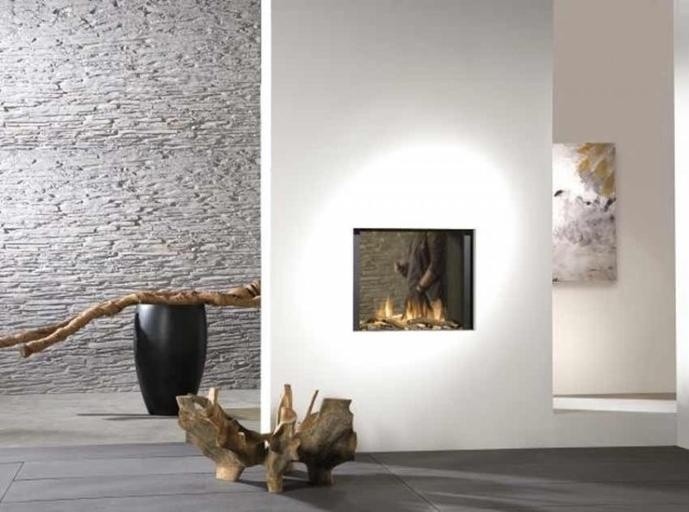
[418, 281, 428, 291]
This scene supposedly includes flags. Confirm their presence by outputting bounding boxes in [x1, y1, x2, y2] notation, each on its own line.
[135, 304, 207, 416]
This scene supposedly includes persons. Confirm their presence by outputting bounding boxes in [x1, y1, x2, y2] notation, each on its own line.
[394, 230, 449, 314]
